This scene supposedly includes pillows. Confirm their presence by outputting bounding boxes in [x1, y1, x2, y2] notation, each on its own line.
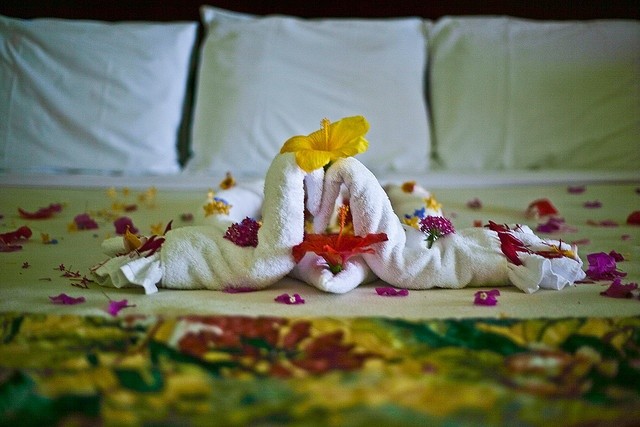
[0, 21, 198, 181]
[431, 18, 638, 185]
[182, 9, 433, 182]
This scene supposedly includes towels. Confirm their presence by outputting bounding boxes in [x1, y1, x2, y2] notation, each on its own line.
[92, 150, 587, 293]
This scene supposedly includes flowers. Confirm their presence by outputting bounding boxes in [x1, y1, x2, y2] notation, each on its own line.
[279, 115, 369, 171]
[292, 206, 388, 265]
